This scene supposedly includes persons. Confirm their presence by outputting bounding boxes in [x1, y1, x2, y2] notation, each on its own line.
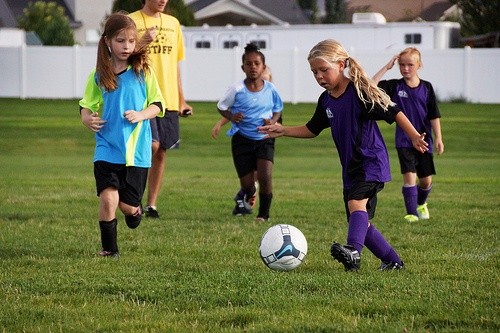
[211, 41, 283, 223]
[79, 11, 165, 258]
[372, 47, 444, 223]
[256, 39, 429, 272]
[125, 0, 193, 218]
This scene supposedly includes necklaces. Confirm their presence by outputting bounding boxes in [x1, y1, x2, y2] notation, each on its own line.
[140, 11, 162, 38]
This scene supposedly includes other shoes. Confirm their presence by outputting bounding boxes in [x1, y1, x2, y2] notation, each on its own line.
[233, 189, 243, 217]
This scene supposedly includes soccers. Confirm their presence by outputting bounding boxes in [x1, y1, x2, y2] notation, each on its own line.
[258, 223, 308, 272]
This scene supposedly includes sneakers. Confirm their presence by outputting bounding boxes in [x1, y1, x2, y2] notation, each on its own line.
[244, 182, 257, 209]
[125, 202, 142, 229]
[331, 243, 361, 271]
[145, 205, 159, 218]
[254, 216, 270, 225]
[404, 215, 418, 222]
[377, 260, 405, 270]
[99, 250, 119, 257]
[416, 201, 430, 219]
